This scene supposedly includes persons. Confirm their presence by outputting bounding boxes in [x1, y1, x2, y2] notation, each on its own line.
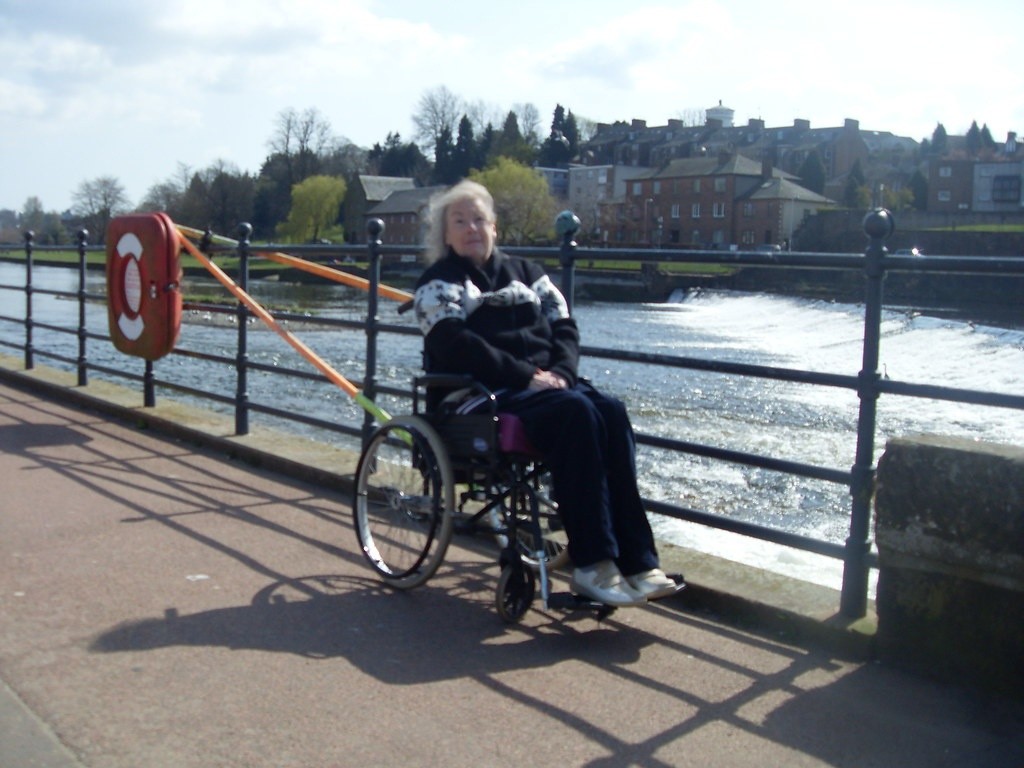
[415, 179, 677, 609]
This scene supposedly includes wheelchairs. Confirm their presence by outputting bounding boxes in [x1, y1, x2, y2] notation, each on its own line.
[350, 299, 686, 622]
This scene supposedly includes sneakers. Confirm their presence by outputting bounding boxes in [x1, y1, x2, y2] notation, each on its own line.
[628, 569, 676, 600]
[569, 560, 647, 607]
